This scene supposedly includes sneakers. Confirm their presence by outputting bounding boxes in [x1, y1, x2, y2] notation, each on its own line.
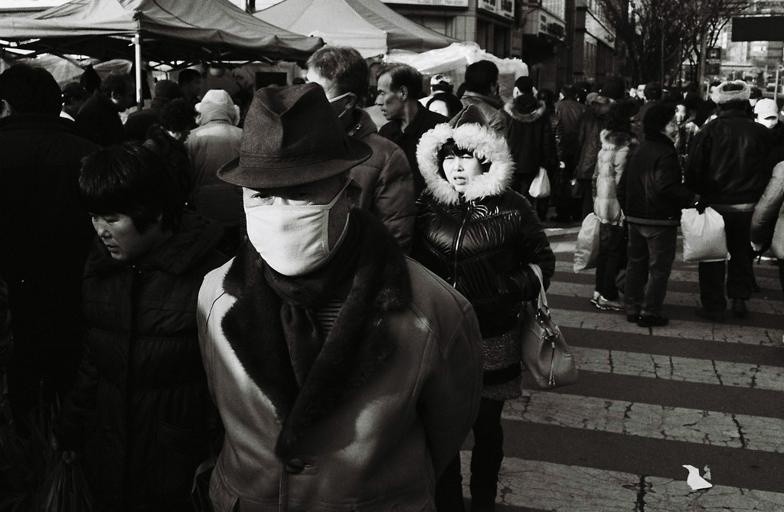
[733, 300, 746, 316]
[591, 292, 668, 327]
[695, 307, 725, 323]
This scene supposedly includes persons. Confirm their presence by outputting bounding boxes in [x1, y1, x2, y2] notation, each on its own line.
[685, 82, 770, 323]
[617, 97, 701, 330]
[0, 66, 99, 510]
[585, 96, 645, 314]
[59, 58, 784, 227]
[750, 158, 784, 294]
[193, 81, 486, 512]
[413, 102, 552, 510]
[67, 140, 227, 510]
[303, 44, 413, 248]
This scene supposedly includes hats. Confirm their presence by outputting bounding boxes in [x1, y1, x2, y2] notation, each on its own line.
[753, 99, 779, 121]
[216, 82, 373, 190]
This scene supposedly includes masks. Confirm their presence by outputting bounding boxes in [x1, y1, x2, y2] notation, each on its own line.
[242, 177, 351, 277]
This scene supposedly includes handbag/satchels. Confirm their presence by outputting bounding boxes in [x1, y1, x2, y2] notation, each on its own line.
[572, 212, 602, 272]
[680, 207, 730, 263]
[521, 308, 576, 391]
